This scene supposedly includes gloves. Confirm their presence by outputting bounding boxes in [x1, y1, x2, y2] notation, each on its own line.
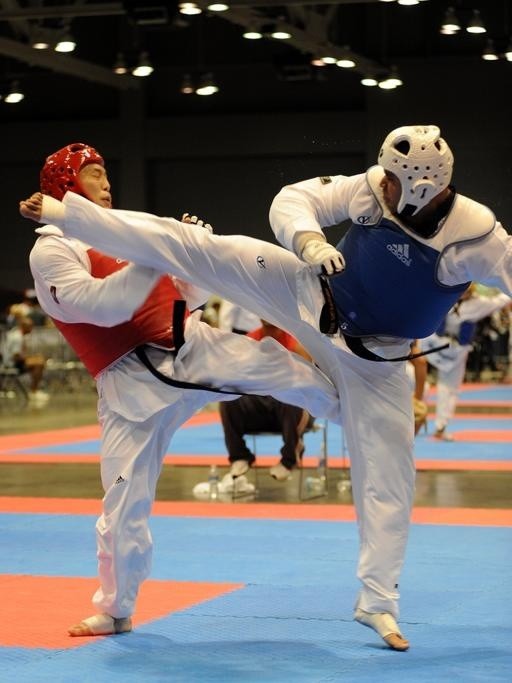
[180, 213, 214, 233]
[302, 239, 346, 277]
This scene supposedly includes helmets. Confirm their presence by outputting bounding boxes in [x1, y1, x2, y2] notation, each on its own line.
[38, 142, 105, 204]
[378, 124, 455, 217]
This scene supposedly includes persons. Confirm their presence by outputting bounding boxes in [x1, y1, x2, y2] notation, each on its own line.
[8, 294, 56, 328]
[28, 142, 427, 637]
[20, 126, 512, 650]
[1, 313, 50, 402]
[204, 295, 316, 481]
[405, 280, 510, 438]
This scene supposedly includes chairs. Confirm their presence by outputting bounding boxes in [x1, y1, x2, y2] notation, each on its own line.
[1, 329, 102, 401]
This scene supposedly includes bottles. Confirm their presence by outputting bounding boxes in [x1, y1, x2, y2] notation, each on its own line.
[209, 464, 218, 497]
[317, 441, 326, 478]
[337, 478, 352, 491]
[304, 476, 325, 492]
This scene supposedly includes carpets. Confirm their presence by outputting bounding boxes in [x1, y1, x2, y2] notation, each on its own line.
[1, 491, 511, 683]
[198, 378, 512, 408]
[1, 412, 511, 477]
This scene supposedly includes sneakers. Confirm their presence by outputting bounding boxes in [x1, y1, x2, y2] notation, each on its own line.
[268, 463, 290, 482]
[230, 460, 250, 479]
